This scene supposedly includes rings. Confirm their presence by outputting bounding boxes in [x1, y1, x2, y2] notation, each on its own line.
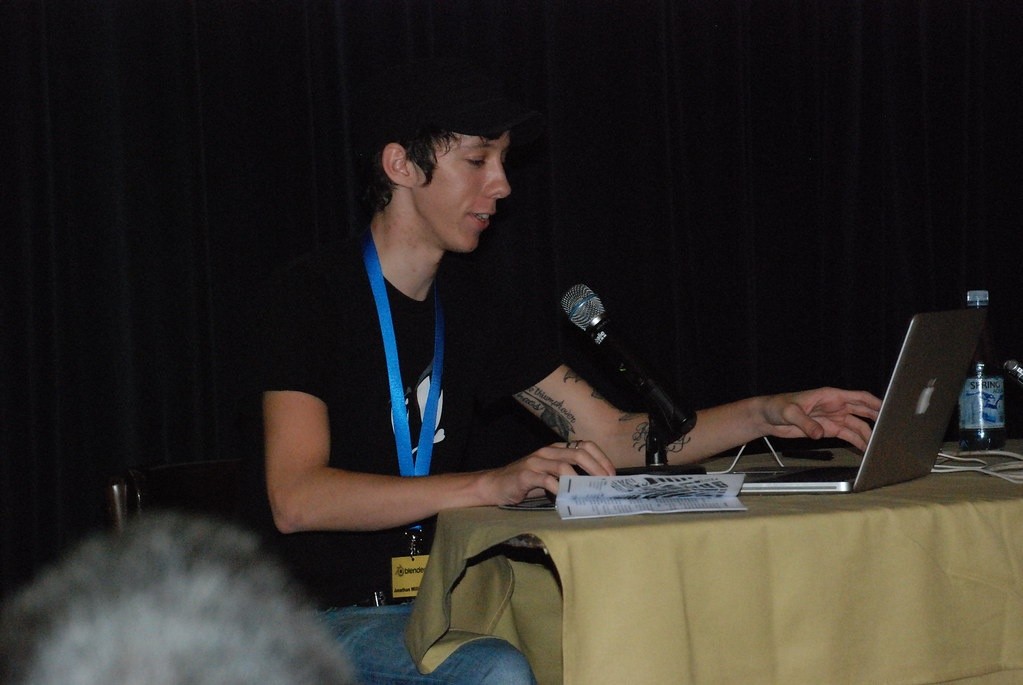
[575, 440, 583, 449]
[567, 440, 578, 449]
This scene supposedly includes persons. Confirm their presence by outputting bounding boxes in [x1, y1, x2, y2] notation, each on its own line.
[239, 82, 880, 685]
[0, 513, 355, 685]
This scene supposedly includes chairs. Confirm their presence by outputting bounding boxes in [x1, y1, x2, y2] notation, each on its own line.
[107, 456, 275, 542]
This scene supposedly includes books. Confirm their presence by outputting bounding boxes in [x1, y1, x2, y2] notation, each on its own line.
[555, 472, 747, 520]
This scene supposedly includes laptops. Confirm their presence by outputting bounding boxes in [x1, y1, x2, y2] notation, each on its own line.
[726, 307, 989, 496]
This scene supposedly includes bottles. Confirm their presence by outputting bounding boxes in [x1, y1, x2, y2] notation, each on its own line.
[960, 291, 1008, 450]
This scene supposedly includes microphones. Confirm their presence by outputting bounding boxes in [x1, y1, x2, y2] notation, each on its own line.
[560, 283, 697, 434]
[1003, 359, 1023, 386]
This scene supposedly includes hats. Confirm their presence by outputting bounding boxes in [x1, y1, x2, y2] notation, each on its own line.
[361, 53, 544, 146]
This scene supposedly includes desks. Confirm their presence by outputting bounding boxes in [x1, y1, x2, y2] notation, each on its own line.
[405, 439, 1023, 685]
